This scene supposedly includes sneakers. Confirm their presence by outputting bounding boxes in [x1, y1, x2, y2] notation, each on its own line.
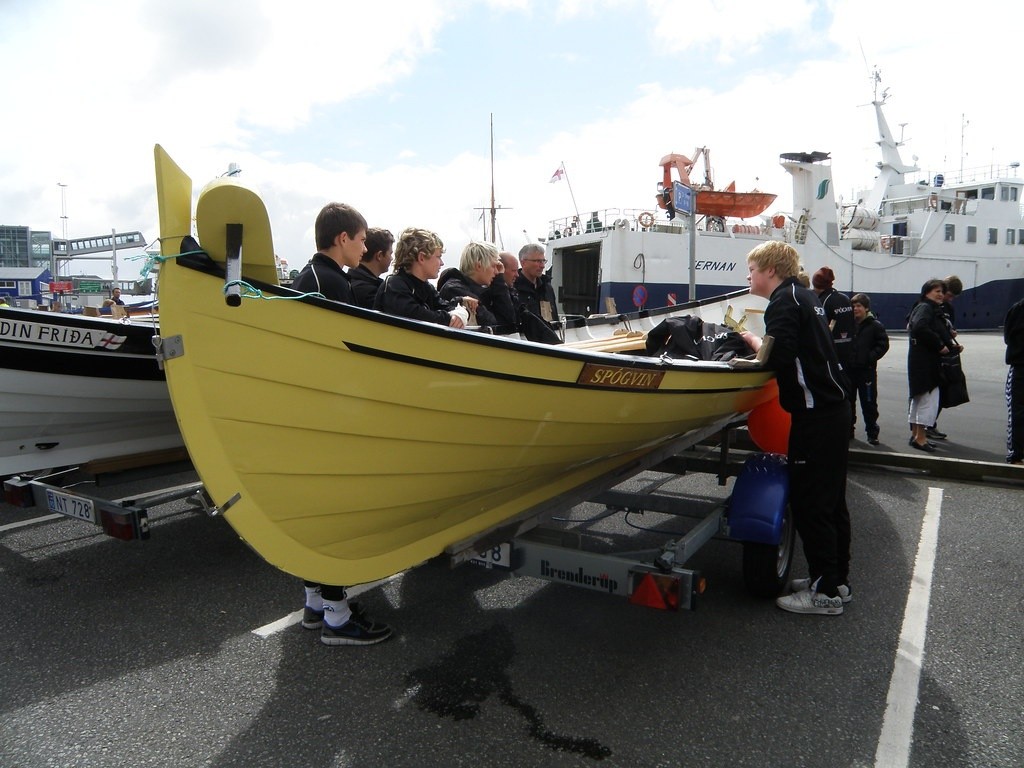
[776, 579, 851, 615]
[302, 603, 395, 646]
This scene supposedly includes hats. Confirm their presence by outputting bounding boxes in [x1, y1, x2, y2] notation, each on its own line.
[813, 267, 835, 288]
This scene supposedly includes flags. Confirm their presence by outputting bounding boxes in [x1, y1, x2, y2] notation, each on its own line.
[551, 164, 564, 184]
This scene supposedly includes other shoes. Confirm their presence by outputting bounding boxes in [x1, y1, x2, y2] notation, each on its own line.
[867, 434, 880, 445]
[926, 429, 947, 439]
[909, 436, 937, 451]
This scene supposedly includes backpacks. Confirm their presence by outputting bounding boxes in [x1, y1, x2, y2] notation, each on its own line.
[647, 315, 757, 361]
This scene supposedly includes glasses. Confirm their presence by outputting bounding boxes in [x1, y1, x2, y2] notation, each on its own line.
[524, 258, 548, 264]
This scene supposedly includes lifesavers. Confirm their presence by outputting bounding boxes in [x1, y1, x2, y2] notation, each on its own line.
[639, 212, 654, 227]
[930, 197, 937, 209]
[881, 234, 894, 250]
[563, 227, 572, 237]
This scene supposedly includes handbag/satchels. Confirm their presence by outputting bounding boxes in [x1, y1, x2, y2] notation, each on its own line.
[938, 365, 970, 409]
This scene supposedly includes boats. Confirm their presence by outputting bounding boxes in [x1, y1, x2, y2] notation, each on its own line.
[154, 143, 780, 585]
[0, 298, 191, 482]
[656, 153, 777, 218]
[542, 66, 1024, 328]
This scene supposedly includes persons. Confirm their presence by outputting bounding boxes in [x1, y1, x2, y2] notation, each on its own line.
[346, 227, 560, 335]
[740, 242, 856, 615]
[0, 297, 9, 307]
[111, 288, 125, 305]
[291, 199, 394, 646]
[799, 265, 1024, 464]
